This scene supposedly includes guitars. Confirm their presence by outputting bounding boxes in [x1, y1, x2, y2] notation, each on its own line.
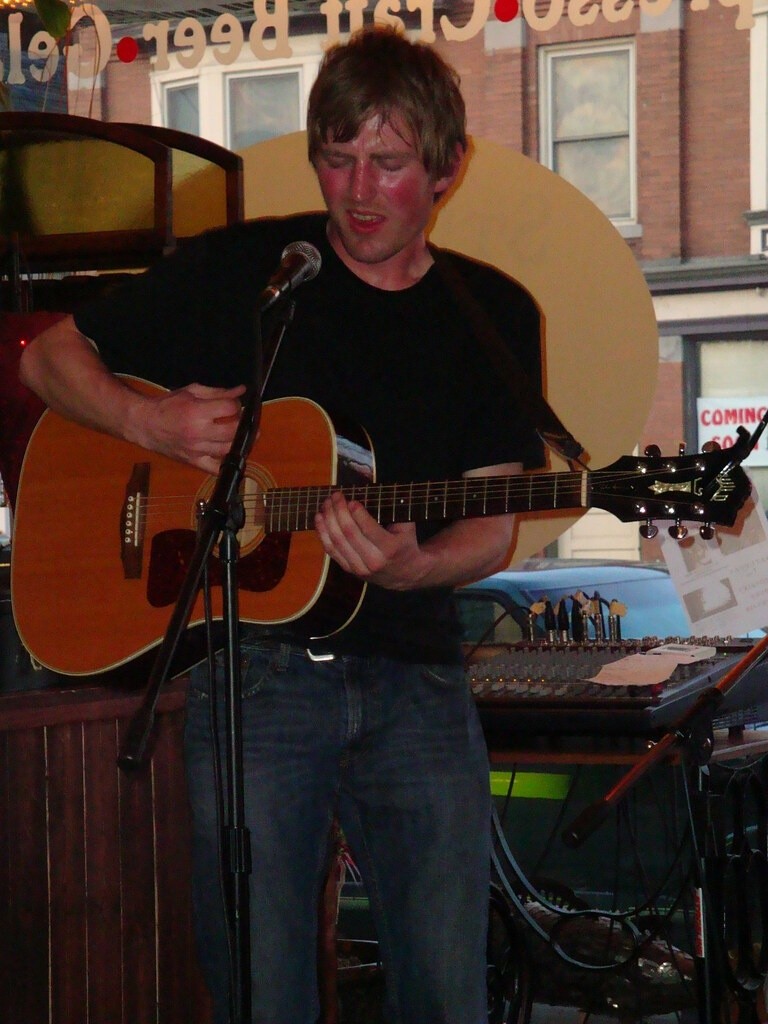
[8, 369, 754, 694]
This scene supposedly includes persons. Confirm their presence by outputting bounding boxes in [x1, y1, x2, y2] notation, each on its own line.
[19, 29, 546, 1024]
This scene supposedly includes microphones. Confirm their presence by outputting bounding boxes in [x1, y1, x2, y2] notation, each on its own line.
[253, 241, 322, 312]
